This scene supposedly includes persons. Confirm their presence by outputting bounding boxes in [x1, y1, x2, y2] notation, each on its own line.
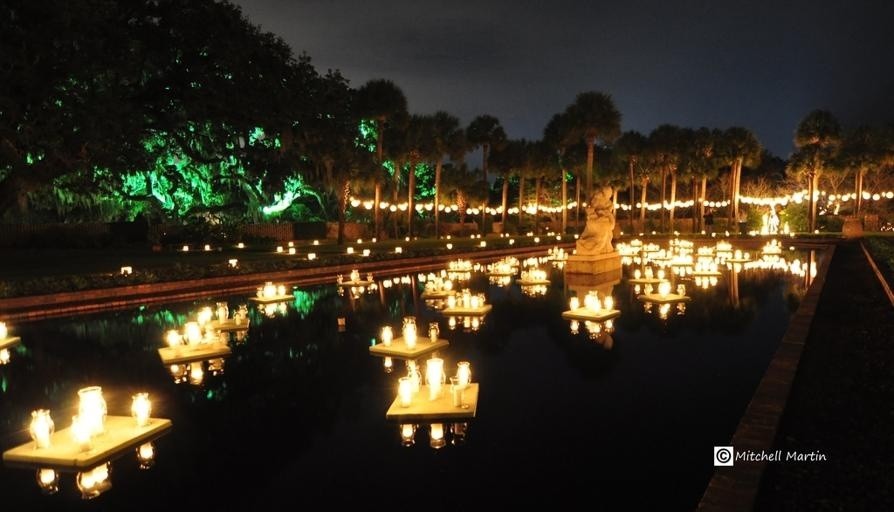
[703, 206, 714, 237]
[576, 186, 616, 254]
[739, 207, 746, 236]
